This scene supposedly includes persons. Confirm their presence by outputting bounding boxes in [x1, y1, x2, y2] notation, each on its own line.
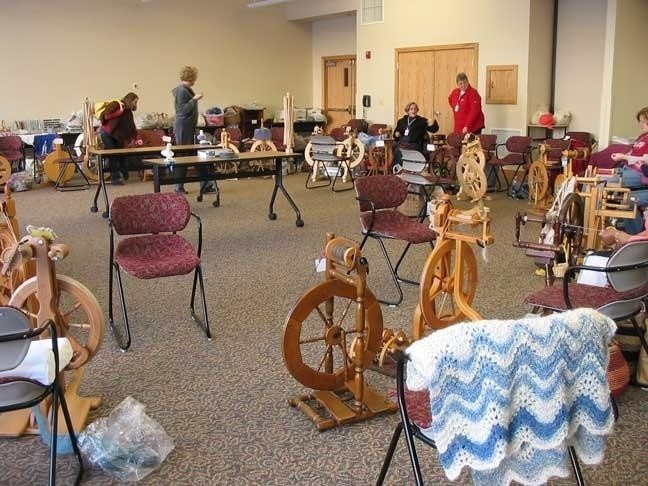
[618, 161, 648, 235]
[598, 216, 648, 249]
[447, 71, 485, 155]
[171, 63, 222, 196]
[97, 91, 140, 187]
[610, 106, 648, 187]
[391, 100, 442, 169]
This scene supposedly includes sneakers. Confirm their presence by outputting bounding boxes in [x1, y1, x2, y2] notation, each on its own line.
[445, 184, 459, 195]
[111, 168, 129, 186]
[174, 186, 189, 194]
[204, 185, 219, 192]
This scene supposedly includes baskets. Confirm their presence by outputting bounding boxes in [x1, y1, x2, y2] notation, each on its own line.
[224, 107, 241, 125]
[552, 244, 569, 277]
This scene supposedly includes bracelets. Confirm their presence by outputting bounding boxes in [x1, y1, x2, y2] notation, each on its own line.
[615, 230, 621, 243]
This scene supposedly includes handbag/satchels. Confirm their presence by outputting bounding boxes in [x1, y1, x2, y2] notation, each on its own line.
[92, 101, 110, 120]
